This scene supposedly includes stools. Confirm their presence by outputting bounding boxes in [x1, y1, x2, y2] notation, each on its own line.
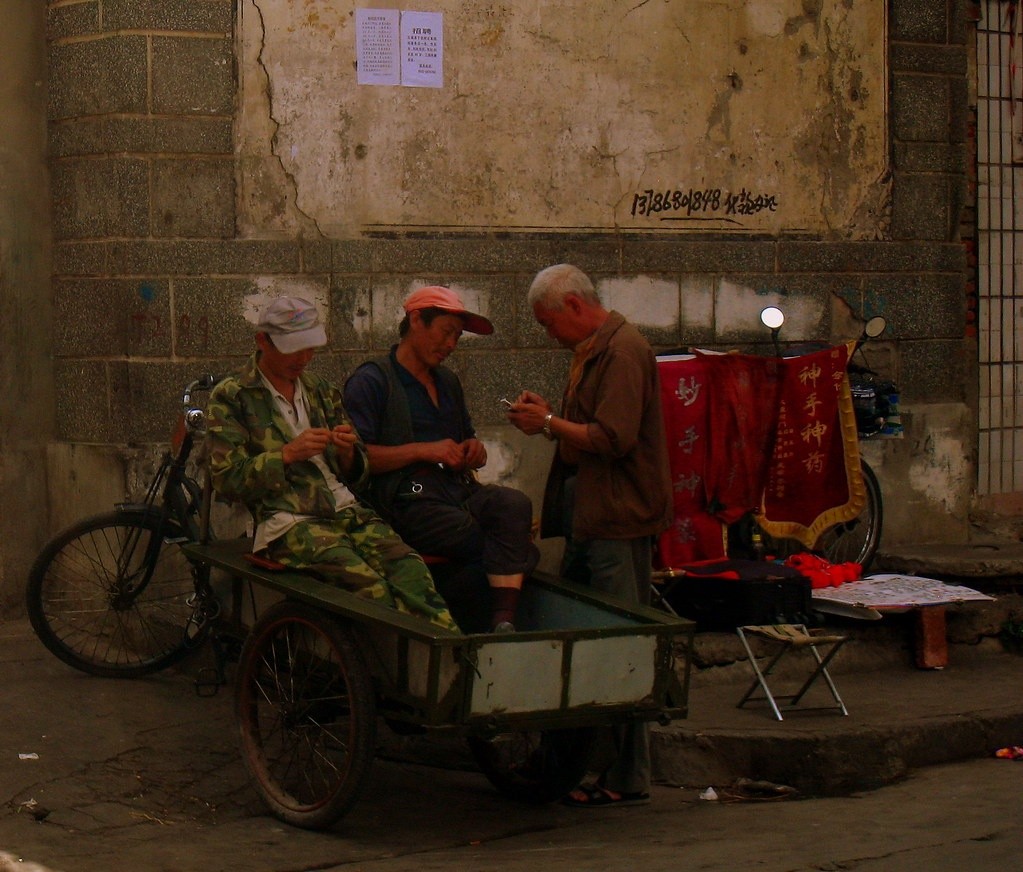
[733, 624, 853, 720]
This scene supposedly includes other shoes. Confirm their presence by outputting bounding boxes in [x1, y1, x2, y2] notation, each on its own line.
[489, 622, 514, 634]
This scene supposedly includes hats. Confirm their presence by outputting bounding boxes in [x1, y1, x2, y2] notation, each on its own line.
[404, 285, 494, 334]
[257, 295, 327, 353]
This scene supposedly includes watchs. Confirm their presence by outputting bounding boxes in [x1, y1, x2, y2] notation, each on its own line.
[542, 414, 556, 442]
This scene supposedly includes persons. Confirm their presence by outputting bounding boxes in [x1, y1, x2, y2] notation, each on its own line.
[205, 296, 475, 660]
[509, 262, 673, 808]
[343, 286, 539, 632]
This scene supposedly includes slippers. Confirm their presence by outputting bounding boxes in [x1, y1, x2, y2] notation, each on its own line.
[562, 782, 651, 807]
[501, 761, 584, 789]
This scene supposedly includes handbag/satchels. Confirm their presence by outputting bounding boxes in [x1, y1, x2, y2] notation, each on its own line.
[847, 346, 904, 441]
